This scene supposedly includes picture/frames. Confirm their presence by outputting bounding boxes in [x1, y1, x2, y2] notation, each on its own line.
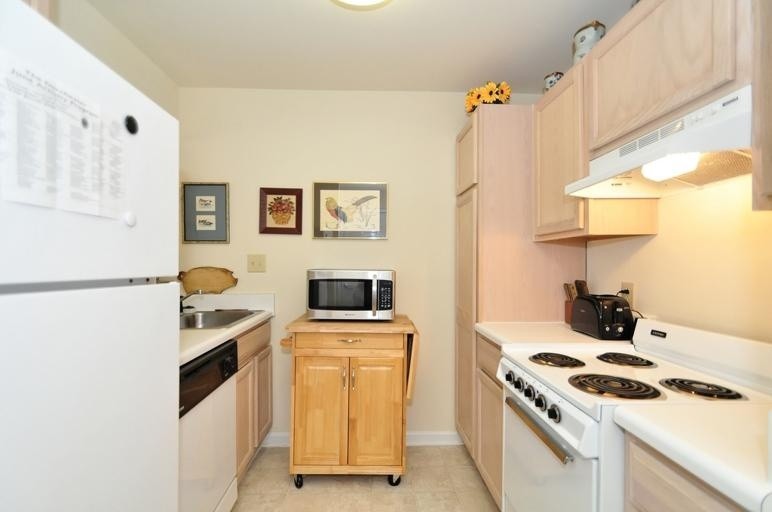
[258, 181, 390, 239]
[180, 179, 231, 246]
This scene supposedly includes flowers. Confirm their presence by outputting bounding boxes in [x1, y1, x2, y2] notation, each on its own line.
[463, 77, 511, 116]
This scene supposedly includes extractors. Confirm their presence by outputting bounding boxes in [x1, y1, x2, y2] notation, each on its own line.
[566, 87, 761, 203]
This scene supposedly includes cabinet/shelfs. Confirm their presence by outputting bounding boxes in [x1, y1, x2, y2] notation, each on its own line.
[453, 188, 479, 456]
[455, 112, 478, 198]
[625, 441, 739, 511]
[293, 333, 405, 467]
[238, 321, 274, 476]
[474, 333, 501, 510]
[532, 63, 584, 237]
[584, 0, 743, 150]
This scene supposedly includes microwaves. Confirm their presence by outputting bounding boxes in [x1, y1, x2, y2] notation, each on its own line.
[305, 269, 397, 322]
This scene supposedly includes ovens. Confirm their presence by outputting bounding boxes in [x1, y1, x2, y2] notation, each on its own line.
[496, 356, 624, 512]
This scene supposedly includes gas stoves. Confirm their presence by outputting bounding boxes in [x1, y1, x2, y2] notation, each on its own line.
[500, 350, 772, 406]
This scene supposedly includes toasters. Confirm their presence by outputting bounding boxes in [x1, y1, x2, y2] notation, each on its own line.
[570, 293, 633, 340]
